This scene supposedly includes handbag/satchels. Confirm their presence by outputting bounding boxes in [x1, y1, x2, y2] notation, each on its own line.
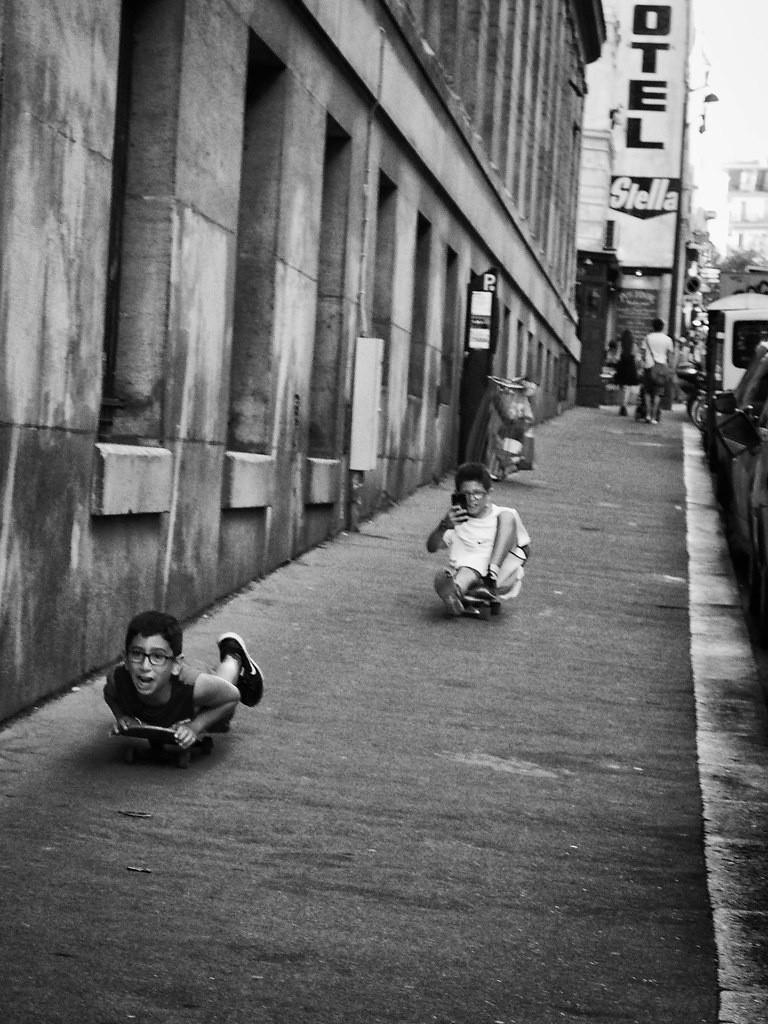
[651, 361, 671, 386]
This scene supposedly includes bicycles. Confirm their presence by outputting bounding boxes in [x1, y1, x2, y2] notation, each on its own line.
[463, 373, 541, 482]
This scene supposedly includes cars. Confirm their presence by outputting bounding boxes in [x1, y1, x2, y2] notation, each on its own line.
[701, 340, 768, 654]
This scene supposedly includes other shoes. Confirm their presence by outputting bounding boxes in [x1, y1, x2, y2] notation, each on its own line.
[651, 419, 658, 424]
[619, 406, 628, 416]
[646, 416, 651, 422]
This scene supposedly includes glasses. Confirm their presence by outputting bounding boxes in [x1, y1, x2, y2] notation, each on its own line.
[466, 492, 487, 500]
[126, 650, 176, 666]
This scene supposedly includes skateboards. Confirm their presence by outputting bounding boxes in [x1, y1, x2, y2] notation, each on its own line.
[111, 718, 213, 768]
[461, 584, 503, 620]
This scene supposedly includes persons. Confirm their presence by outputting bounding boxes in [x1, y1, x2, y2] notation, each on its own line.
[605, 318, 704, 426]
[610, 107, 625, 172]
[102, 611, 266, 748]
[423, 464, 531, 617]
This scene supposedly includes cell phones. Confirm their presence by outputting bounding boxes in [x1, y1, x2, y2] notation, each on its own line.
[451, 493, 468, 522]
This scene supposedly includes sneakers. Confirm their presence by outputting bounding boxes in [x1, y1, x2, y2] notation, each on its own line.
[436, 568, 464, 617]
[217, 631, 263, 707]
[474, 576, 498, 598]
